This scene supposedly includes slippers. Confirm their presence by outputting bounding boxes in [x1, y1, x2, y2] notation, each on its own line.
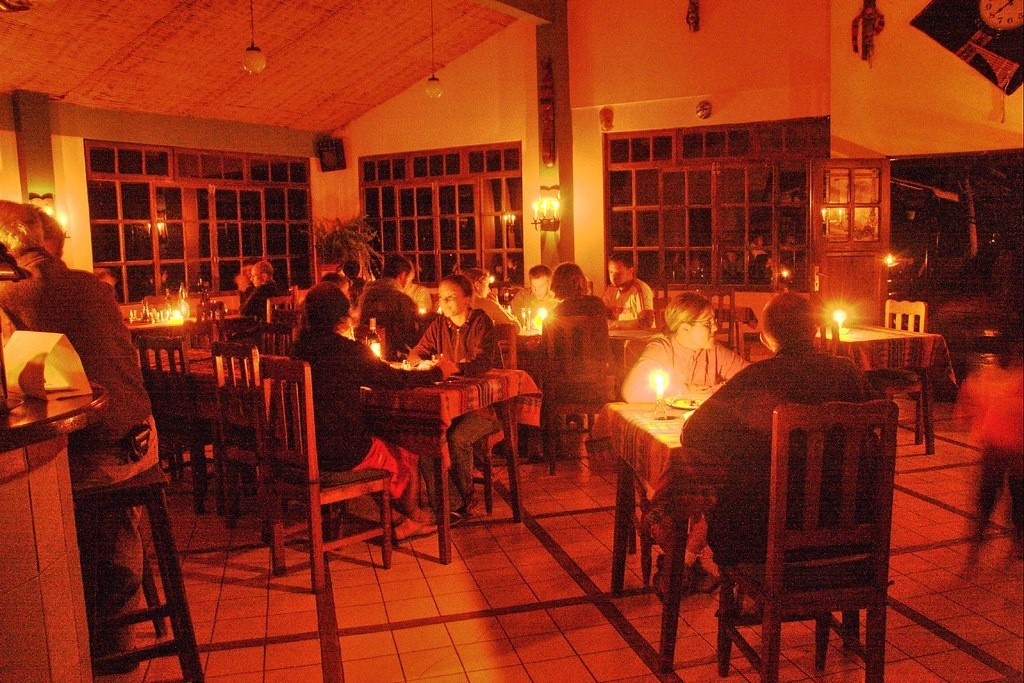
[441, 510, 477, 527]
[394, 523, 439, 545]
[409, 509, 438, 526]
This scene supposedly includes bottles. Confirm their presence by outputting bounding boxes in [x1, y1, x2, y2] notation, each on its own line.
[503, 291, 512, 315]
[365, 317, 382, 361]
[130, 282, 191, 324]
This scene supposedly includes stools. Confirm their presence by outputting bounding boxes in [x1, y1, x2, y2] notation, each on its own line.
[74, 462, 209, 683]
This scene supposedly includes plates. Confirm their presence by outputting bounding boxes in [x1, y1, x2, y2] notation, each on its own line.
[666, 395, 705, 410]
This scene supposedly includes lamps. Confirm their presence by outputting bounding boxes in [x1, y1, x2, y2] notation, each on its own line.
[422, 0, 447, 102]
[241, 0, 270, 76]
[529, 193, 566, 232]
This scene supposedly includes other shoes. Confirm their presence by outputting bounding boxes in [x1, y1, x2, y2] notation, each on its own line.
[691, 558, 720, 593]
[91, 646, 141, 674]
[95, 566, 141, 618]
[652, 553, 665, 602]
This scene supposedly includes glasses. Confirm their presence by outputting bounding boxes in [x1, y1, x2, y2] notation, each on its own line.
[686, 315, 718, 329]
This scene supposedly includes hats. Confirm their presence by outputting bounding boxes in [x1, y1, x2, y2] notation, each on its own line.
[305, 282, 350, 330]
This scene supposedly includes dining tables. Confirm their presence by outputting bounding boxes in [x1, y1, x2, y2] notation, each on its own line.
[820, 320, 962, 453]
[359, 355, 546, 567]
[131, 334, 216, 457]
[713, 301, 763, 355]
[168, 359, 272, 530]
[518, 319, 673, 464]
[195, 299, 232, 330]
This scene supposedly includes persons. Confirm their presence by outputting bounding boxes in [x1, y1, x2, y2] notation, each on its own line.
[678, 289, 893, 619]
[281, 252, 657, 542]
[948, 326, 1024, 582]
[721, 233, 802, 283]
[518, 261, 625, 459]
[619, 291, 759, 610]
[98, 280, 132, 346]
[405, 272, 510, 524]
[601, 252, 657, 363]
[225, 260, 283, 341]
[91, 267, 120, 288]
[1, 199, 161, 678]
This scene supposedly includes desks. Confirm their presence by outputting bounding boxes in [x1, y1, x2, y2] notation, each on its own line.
[586, 393, 734, 675]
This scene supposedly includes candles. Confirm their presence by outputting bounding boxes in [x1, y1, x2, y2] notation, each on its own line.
[654, 375, 666, 399]
[837, 310, 844, 330]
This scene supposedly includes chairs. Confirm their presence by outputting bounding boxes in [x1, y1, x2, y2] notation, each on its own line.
[619, 335, 721, 595]
[435, 318, 526, 514]
[262, 294, 296, 345]
[698, 286, 738, 350]
[206, 341, 339, 541]
[537, 310, 614, 479]
[139, 328, 217, 504]
[248, 353, 397, 598]
[710, 398, 902, 683]
[867, 297, 933, 445]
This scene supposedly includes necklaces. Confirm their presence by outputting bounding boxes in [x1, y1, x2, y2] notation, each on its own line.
[443, 306, 471, 332]
